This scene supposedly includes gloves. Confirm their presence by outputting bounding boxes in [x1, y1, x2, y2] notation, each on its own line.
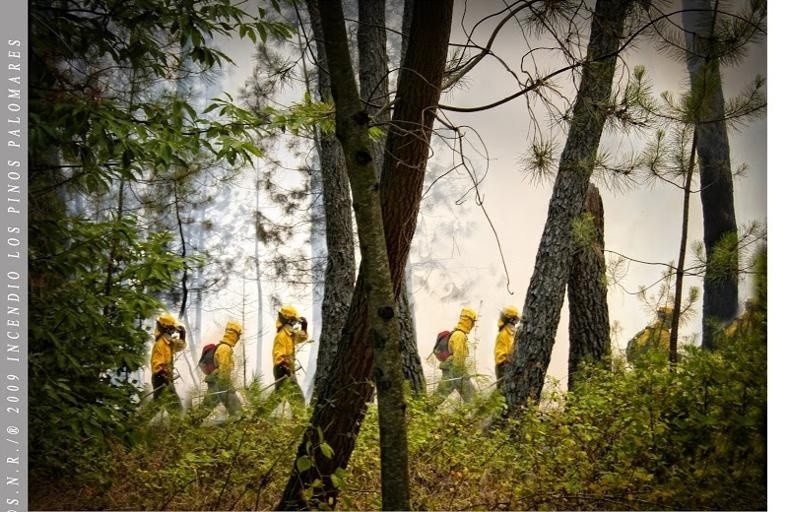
[278, 363, 292, 377]
[300, 316, 307, 331]
[177, 325, 186, 341]
[156, 369, 171, 385]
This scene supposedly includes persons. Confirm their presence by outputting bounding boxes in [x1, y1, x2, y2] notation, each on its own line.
[265, 306, 309, 419]
[142, 315, 183, 417]
[495, 306, 520, 388]
[626, 306, 674, 374]
[429, 308, 478, 409]
[195, 320, 244, 420]
[727, 297, 755, 336]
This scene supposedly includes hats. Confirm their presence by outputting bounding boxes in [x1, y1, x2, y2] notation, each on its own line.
[275, 306, 303, 329]
[154, 315, 182, 342]
[457, 308, 479, 334]
[497, 306, 519, 330]
[219, 321, 243, 347]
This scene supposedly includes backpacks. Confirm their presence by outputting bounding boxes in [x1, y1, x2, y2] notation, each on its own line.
[199, 343, 217, 374]
[433, 331, 451, 360]
